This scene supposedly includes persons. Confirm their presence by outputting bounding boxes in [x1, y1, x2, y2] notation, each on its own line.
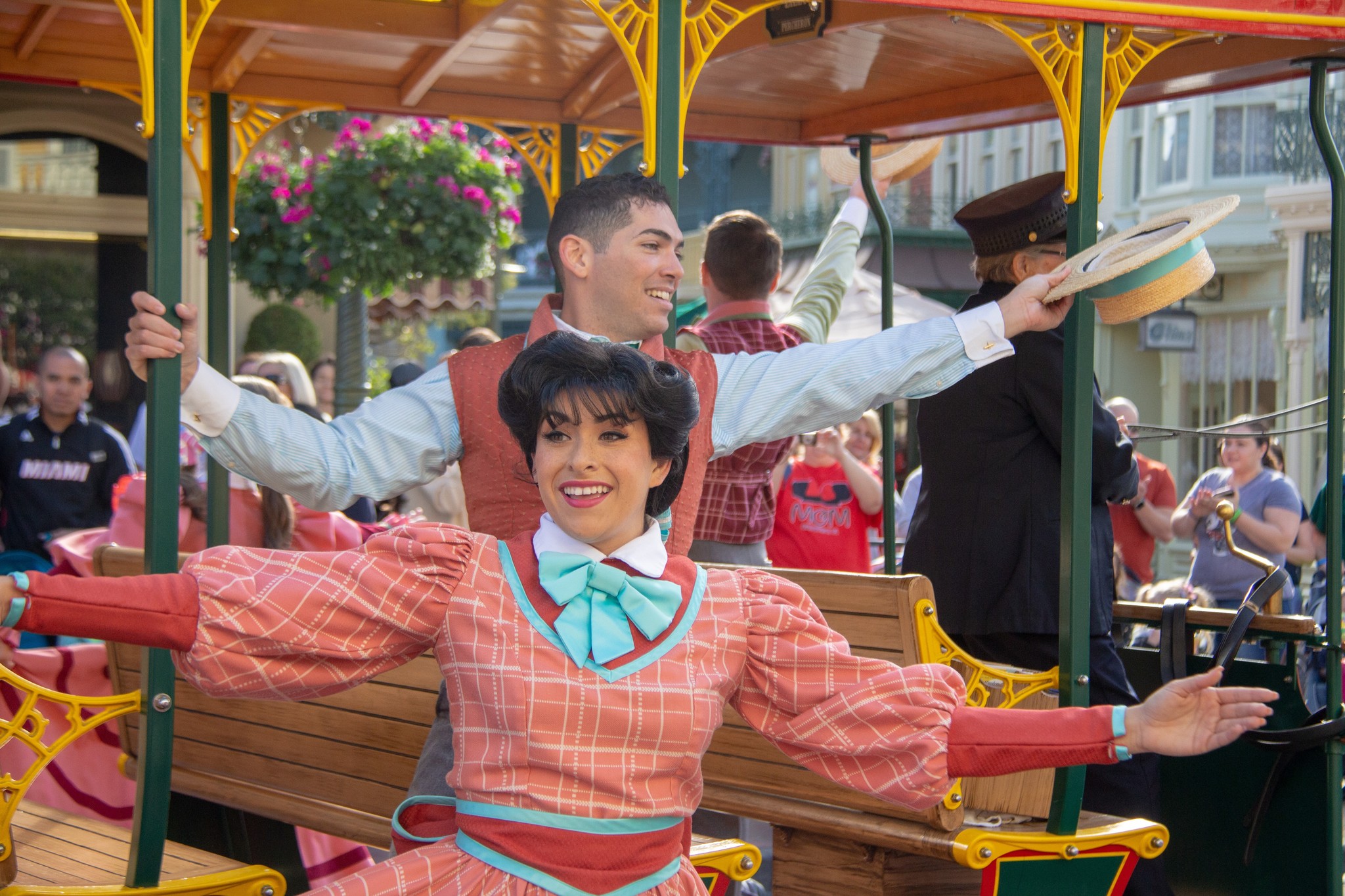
[124, 172, 1077, 859]
[0, 331, 1279, 896]
[768, 400, 923, 579]
[0, 326, 502, 646]
[900, 173, 1174, 896]
[1102, 396, 1345, 722]
[677, 171, 895, 565]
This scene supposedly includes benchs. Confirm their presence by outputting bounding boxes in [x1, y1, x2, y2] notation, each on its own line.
[0, 662, 288, 896]
[91, 540, 1170, 896]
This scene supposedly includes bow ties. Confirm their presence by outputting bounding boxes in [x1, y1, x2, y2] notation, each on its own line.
[537, 550, 683, 670]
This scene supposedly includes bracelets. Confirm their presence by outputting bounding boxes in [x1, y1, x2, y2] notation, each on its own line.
[1188, 509, 1197, 519]
[1318, 558, 1327, 566]
[1230, 509, 1241, 524]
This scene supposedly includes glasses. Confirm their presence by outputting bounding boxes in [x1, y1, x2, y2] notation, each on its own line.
[267, 375, 286, 384]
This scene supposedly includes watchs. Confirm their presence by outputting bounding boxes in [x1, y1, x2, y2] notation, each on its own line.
[1134, 498, 1145, 509]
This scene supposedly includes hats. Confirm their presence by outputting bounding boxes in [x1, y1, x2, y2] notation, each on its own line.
[820, 137, 945, 187]
[1043, 194, 1241, 325]
[952, 172, 1105, 258]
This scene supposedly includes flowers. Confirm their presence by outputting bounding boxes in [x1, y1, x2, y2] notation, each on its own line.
[196, 113, 524, 306]
